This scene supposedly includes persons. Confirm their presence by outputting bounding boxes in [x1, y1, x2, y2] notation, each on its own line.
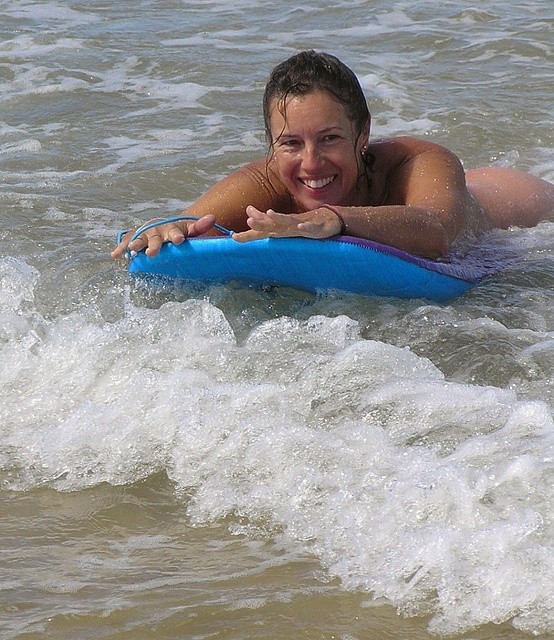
[109, 52, 554, 260]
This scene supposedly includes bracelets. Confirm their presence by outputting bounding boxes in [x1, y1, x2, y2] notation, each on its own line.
[318, 205, 348, 237]
[134, 217, 167, 233]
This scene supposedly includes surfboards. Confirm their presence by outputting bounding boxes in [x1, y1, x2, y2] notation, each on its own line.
[127, 235, 518, 302]
